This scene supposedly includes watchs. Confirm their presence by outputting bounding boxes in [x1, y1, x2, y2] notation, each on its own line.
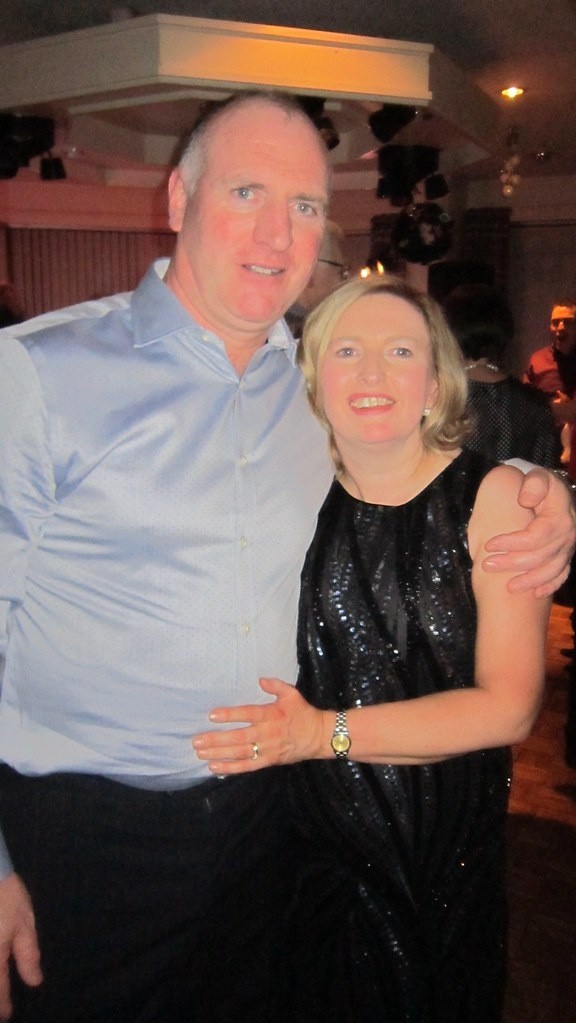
[332, 711, 351, 760]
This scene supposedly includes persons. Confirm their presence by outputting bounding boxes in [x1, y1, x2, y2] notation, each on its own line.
[195, 268, 561, 1022]
[0, 89, 575, 1022]
[441, 281, 559, 471]
[525, 297, 576, 487]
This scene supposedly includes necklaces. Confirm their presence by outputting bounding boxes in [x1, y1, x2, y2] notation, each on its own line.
[464, 363, 500, 372]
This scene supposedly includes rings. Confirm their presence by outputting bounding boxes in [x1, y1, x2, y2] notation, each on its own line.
[248, 743, 259, 760]
